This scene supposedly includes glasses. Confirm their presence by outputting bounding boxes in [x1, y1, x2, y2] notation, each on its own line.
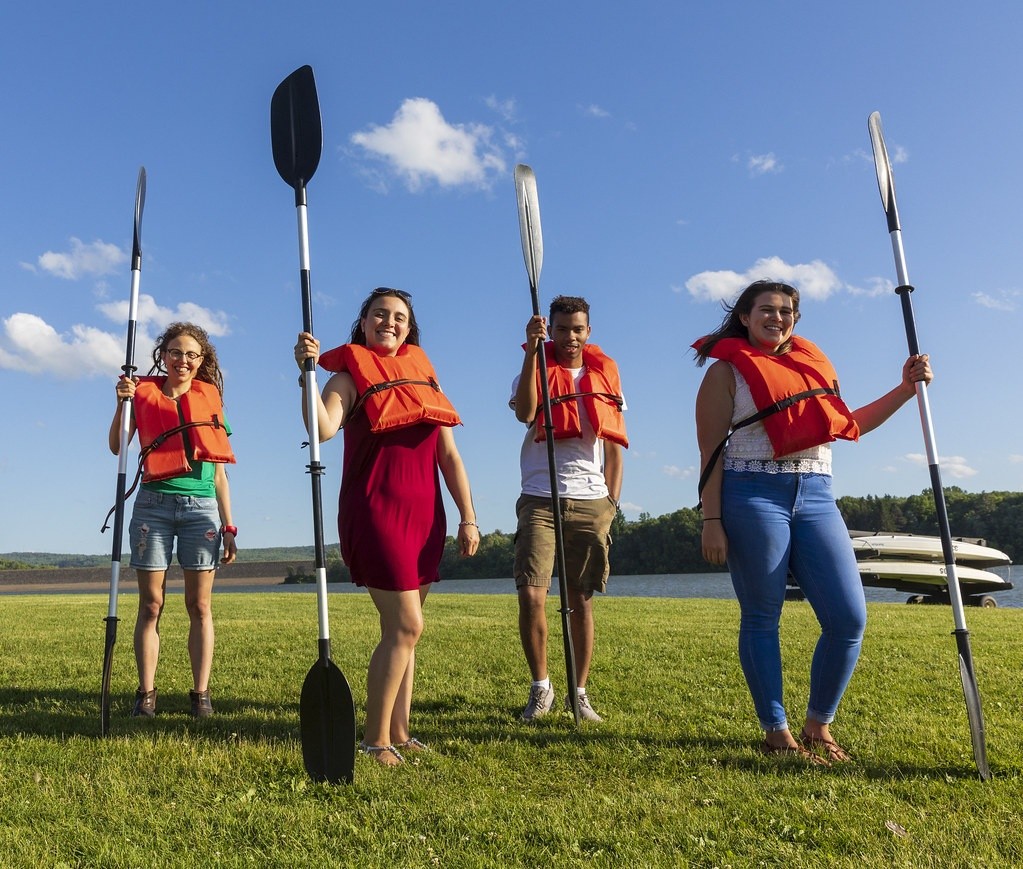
[163, 348, 204, 359]
[372, 287, 413, 309]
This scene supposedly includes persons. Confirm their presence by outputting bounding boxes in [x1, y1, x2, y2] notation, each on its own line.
[508, 296, 628, 723]
[108, 322, 237, 719]
[293, 287, 480, 768]
[695, 281, 934, 766]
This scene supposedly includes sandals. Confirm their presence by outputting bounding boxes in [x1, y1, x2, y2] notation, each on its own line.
[361, 742, 405, 768]
[391, 737, 431, 752]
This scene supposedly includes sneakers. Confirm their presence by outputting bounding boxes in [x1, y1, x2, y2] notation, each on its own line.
[565, 693, 603, 720]
[132, 685, 157, 717]
[190, 689, 215, 715]
[524, 683, 555, 722]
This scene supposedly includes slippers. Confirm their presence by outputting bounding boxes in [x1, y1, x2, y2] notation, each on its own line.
[762, 738, 832, 767]
[798, 726, 851, 763]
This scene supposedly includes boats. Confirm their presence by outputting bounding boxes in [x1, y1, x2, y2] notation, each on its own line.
[847, 537, 1012, 586]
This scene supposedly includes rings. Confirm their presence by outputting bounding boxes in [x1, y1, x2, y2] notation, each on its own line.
[301, 347, 303, 353]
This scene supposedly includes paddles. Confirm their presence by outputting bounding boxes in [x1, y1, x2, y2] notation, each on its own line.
[866, 110, 994, 784]
[270, 64, 357, 784]
[99, 165, 148, 737]
[513, 163, 581, 730]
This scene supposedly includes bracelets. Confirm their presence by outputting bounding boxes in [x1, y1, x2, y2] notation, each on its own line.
[458, 521, 479, 528]
[298, 375, 317, 388]
[702, 518, 722, 521]
[221, 526, 238, 537]
